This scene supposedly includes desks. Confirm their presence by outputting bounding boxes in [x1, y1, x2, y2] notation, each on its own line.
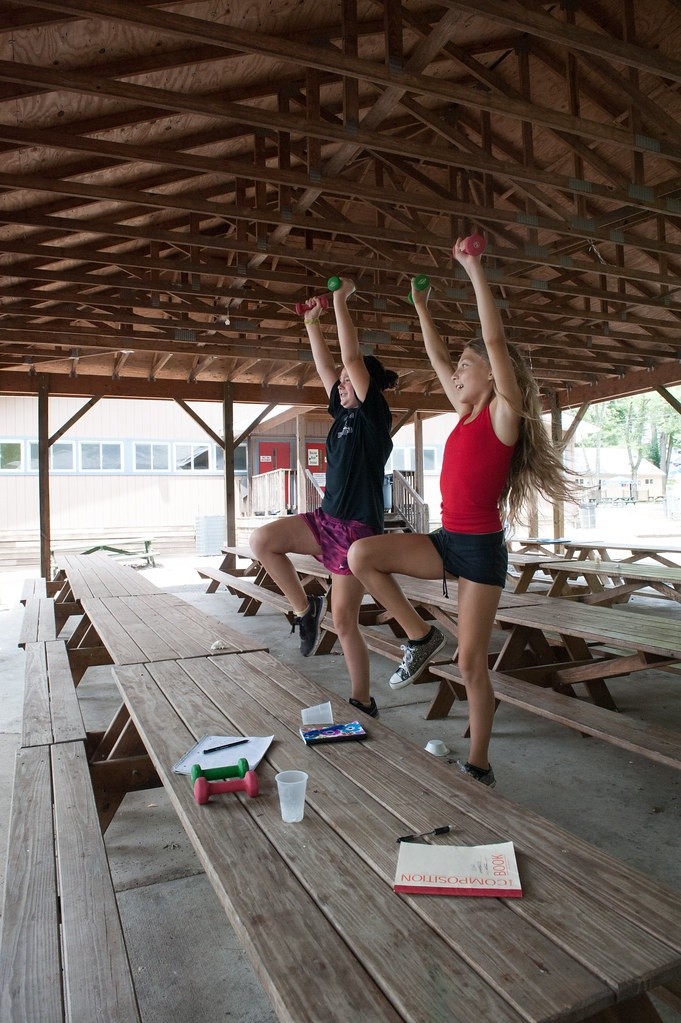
[563, 542, 681, 569]
[65, 593, 271, 688]
[506, 537, 570, 558]
[53, 554, 165, 639]
[312, 571, 577, 720]
[87, 652, 681, 1023]
[463, 603, 681, 741]
[52, 536, 158, 550]
[504, 553, 576, 595]
[197, 545, 335, 631]
[538, 561, 681, 617]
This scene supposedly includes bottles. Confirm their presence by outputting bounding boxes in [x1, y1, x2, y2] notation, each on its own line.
[274, 771, 308, 823]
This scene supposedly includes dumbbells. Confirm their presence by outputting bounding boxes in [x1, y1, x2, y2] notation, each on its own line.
[408, 274, 430, 305]
[191, 758, 260, 805]
[296, 296, 328, 317]
[452, 235, 486, 256]
[327, 276, 341, 291]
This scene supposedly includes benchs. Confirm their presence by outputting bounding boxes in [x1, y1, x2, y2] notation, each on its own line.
[108, 547, 162, 560]
[1, 569, 165, 1023]
[194, 567, 681, 800]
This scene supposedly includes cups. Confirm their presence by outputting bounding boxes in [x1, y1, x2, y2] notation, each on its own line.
[301, 701, 334, 725]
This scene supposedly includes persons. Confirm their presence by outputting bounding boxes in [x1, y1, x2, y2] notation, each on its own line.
[249, 277, 401, 720]
[346, 234, 600, 789]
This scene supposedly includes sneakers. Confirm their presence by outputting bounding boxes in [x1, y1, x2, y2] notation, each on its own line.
[457, 759, 495, 789]
[290, 595, 328, 657]
[349, 696, 379, 720]
[389, 626, 446, 690]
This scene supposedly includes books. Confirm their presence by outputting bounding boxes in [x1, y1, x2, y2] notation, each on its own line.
[299, 720, 368, 745]
[394, 841, 523, 897]
[170, 733, 275, 776]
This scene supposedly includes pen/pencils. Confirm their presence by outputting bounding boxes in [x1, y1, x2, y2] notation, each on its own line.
[203, 740, 249, 754]
[396, 824, 456, 844]
[304, 724, 344, 736]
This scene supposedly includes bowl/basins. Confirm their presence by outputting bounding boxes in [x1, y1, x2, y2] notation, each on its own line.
[424, 740, 450, 757]
